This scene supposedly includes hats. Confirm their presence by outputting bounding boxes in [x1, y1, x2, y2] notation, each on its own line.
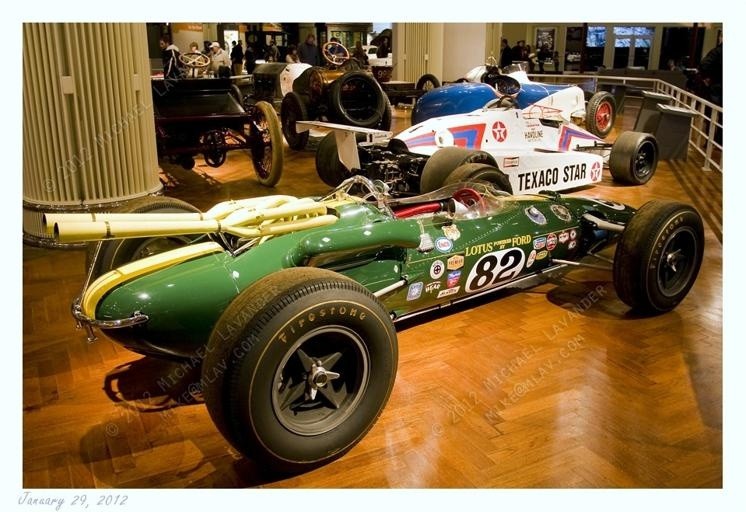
[208, 42, 220, 49]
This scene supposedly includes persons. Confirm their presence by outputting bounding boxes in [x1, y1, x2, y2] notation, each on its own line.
[159, 33, 390, 76]
[667, 60, 682, 71]
[688, 43, 722, 149]
[501, 38, 553, 74]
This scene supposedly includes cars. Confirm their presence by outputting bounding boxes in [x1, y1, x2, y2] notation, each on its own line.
[151, 41, 391, 187]
